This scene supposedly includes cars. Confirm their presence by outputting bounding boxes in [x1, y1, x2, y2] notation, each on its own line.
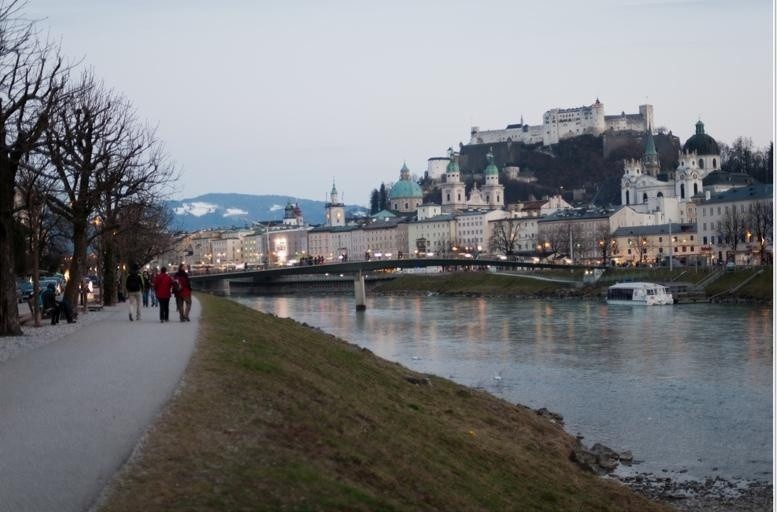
[15, 272, 101, 325]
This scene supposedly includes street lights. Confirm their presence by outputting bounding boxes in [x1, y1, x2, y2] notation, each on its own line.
[250, 220, 271, 265]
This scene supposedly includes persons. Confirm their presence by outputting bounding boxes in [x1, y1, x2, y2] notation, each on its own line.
[125, 260, 194, 323]
[37, 283, 77, 326]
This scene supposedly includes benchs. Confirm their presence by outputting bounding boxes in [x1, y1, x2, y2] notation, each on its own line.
[27, 296, 74, 322]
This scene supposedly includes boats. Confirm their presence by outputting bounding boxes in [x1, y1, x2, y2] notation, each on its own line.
[601, 282, 675, 307]
